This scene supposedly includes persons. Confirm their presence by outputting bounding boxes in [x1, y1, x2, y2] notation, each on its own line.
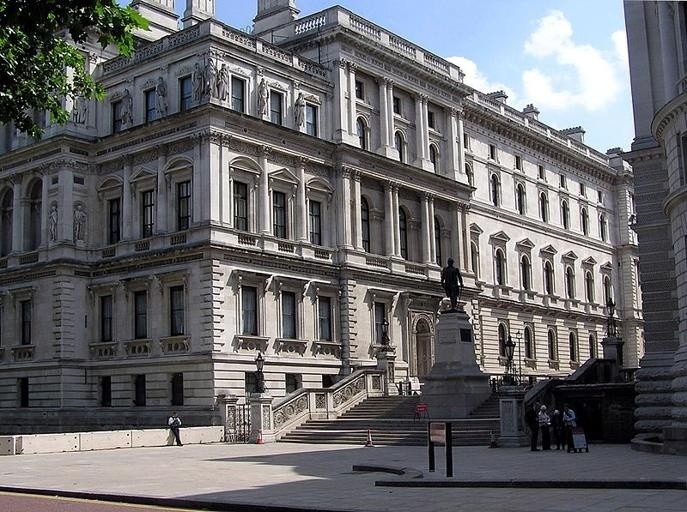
[168, 411, 184, 446]
[525, 403, 577, 453]
[441, 258, 463, 309]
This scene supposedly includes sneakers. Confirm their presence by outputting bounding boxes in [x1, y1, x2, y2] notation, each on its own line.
[531, 447, 565, 452]
[177, 444, 183, 446]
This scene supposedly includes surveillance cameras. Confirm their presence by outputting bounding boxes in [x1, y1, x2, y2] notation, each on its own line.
[629, 215, 635, 222]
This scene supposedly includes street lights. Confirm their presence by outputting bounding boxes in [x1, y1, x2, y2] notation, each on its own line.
[253, 351, 265, 393]
[515, 331, 523, 384]
[605, 298, 617, 336]
[503, 334, 516, 385]
[381, 319, 390, 345]
